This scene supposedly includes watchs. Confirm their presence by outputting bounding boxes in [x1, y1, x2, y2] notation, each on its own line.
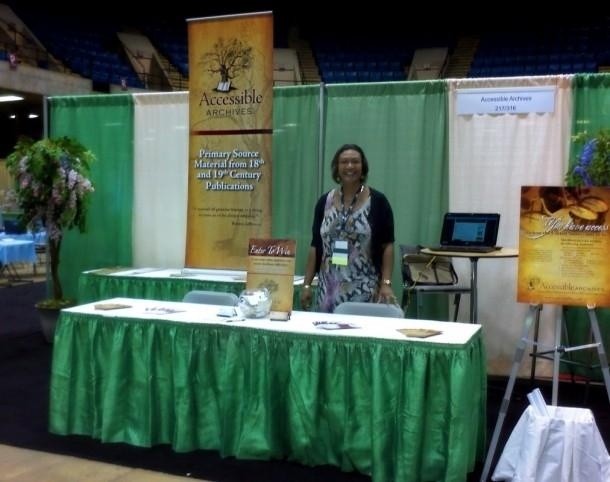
[379, 278, 392, 286]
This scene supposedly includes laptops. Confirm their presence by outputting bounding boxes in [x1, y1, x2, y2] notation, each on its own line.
[429, 211, 501, 253]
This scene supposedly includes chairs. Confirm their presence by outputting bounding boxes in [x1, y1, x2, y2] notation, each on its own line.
[181, 290, 240, 307]
[333, 301, 405, 319]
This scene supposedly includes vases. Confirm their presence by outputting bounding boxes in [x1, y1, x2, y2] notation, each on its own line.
[33, 299, 79, 344]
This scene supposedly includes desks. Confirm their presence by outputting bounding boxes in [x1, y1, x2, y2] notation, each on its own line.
[47, 298, 488, 482]
[77, 267, 319, 312]
[420, 248, 519, 324]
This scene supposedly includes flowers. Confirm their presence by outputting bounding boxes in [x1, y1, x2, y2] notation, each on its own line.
[565, 119, 610, 188]
[5, 136, 97, 302]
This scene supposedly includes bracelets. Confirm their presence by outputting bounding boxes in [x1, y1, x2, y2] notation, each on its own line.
[302, 283, 311, 289]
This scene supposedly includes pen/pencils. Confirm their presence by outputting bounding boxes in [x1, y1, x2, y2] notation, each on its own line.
[226, 319, 245, 322]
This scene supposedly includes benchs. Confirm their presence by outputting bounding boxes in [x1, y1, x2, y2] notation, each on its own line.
[398, 243, 471, 319]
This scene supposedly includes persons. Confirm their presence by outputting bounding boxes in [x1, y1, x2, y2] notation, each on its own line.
[299, 143, 404, 315]
